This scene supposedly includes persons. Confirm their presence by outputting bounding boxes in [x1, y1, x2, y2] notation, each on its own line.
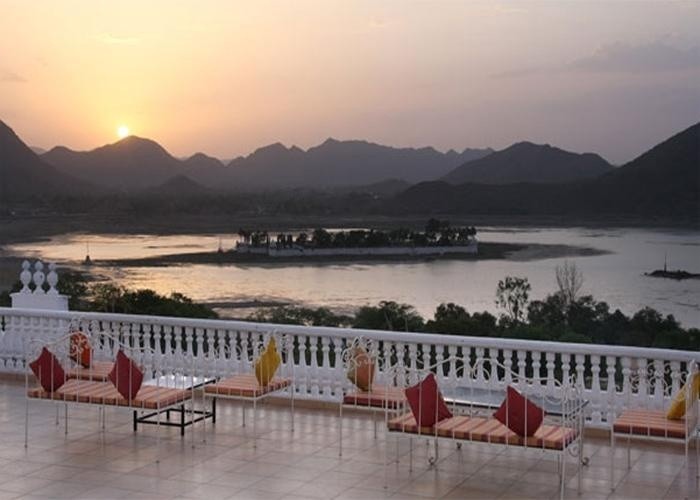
[248, 227, 477, 248]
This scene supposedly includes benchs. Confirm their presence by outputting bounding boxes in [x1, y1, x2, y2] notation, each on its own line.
[382, 353, 590, 500]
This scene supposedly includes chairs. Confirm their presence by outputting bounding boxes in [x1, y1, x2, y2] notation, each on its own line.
[19, 316, 298, 458]
[334, 337, 430, 455]
[606, 359, 700, 493]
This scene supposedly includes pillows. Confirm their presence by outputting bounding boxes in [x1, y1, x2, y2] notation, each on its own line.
[347, 348, 377, 389]
[666, 371, 699, 418]
[401, 373, 451, 426]
[491, 385, 546, 438]
